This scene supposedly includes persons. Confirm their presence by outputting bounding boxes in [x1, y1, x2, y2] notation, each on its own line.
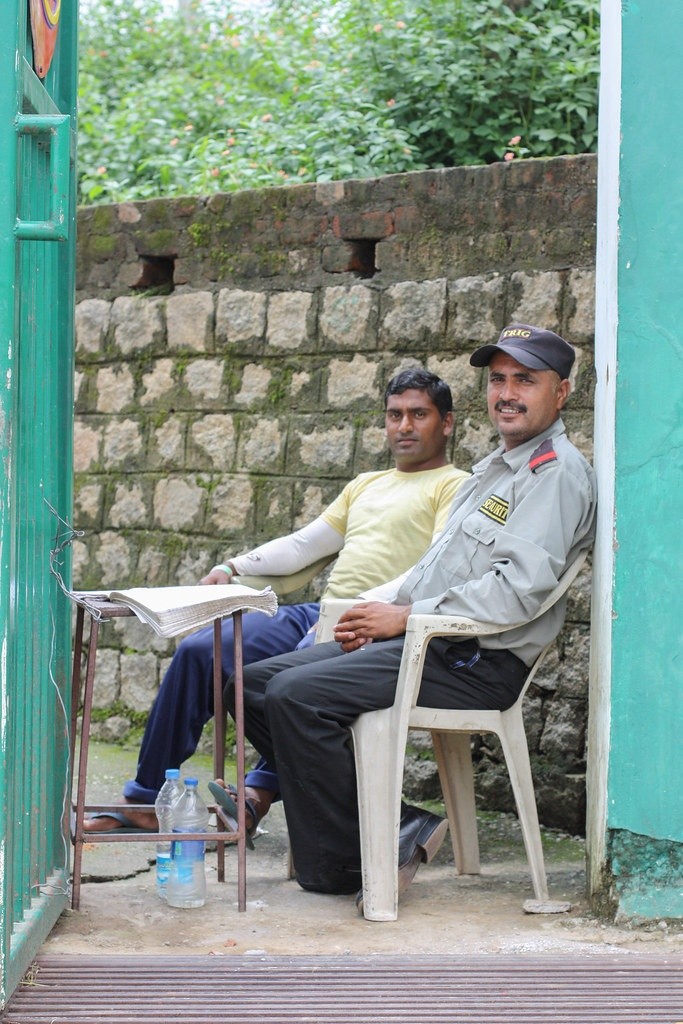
[222, 325, 599, 917]
[76, 368, 475, 851]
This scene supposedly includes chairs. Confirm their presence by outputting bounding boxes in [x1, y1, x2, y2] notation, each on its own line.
[286, 550, 590, 923]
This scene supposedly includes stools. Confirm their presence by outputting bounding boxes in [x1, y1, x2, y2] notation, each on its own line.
[72, 599, 246, 914]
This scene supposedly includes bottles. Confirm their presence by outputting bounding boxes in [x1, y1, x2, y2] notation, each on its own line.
[167, 778, 207, 909]
[154, 769, 185, 900]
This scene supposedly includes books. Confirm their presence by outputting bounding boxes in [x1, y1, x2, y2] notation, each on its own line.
[70, 583, 277, 637]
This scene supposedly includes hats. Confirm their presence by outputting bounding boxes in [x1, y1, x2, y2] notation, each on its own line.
[470, 324, 576, 379]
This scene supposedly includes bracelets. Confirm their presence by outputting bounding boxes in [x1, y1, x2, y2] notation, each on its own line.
[210, 565, 233, 577]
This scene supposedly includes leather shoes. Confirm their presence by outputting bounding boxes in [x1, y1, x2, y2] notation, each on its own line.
[356, 805, 449, 917]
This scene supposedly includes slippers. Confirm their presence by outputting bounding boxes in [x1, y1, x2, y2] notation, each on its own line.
[84, 811, 159, 834]
[208, 780, 258, 851]
